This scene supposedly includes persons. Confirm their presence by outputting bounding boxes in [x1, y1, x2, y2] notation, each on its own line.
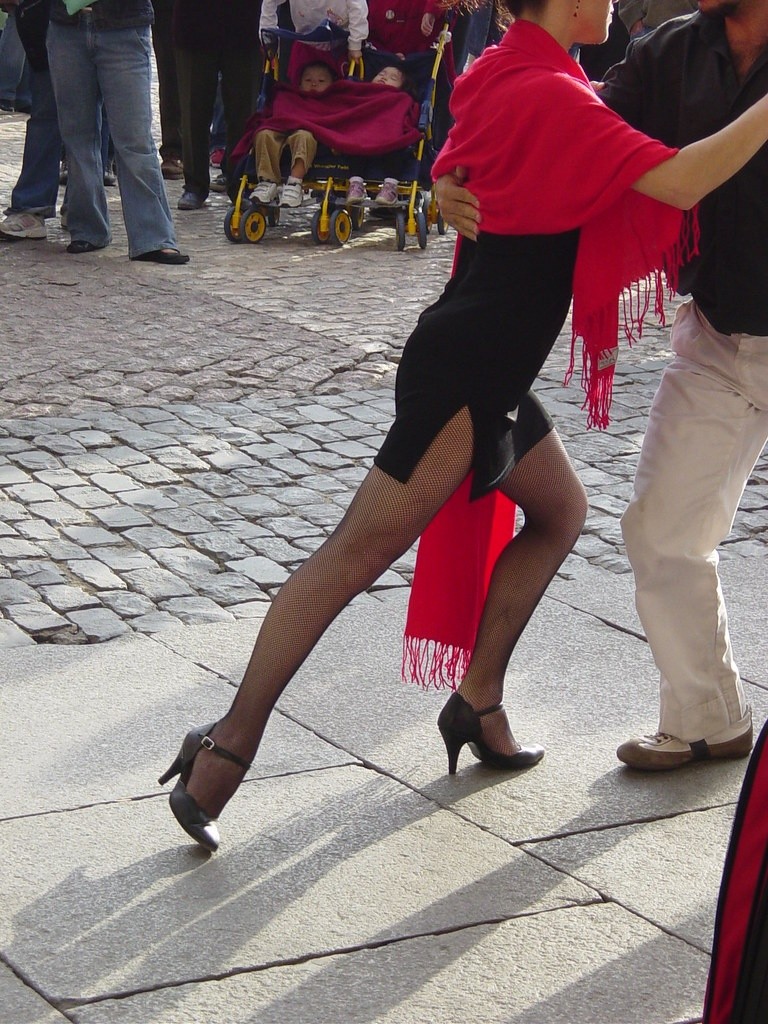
[156, 0, 768, 854]
[0, 0, 699, 265]
[434, 0, 768, 773]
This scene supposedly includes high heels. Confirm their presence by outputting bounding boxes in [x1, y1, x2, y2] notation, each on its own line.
[438, 692, 546, 775]
[159, 723, 251, 852]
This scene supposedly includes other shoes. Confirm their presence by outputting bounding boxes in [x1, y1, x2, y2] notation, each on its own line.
[59, 159, 69, 184]
[132, 248, 190, 264]
[210, 174, 230, 192]
[67, 240, 96, 252]
[0, 100, 14, 113]
[177, 191, 202, 209]
[103, 166, 117, 186]
[161, 158, 184, 179]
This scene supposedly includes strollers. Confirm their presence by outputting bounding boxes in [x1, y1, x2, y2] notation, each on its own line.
[222, 7, 457, 253]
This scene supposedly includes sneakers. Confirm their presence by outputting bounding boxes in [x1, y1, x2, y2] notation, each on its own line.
[616, 706, 754, 771]
[61, 216, 67, 228]
[249, 181, 278, 203]
[279, 182, 303, 208]
[208, 149, 225, 167]
[375, 182, 398, 205]
[346, 181, 367, 203]
[0, 212, 47, 239]
